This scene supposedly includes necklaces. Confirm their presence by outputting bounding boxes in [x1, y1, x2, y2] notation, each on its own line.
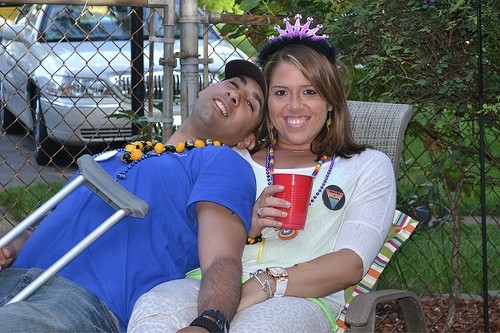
[266, 138, 335, 206]
[92, 141, 225, 182]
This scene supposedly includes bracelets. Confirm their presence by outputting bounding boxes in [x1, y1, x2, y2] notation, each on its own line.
[246, 234, 262, 245]
[249, 269, 272, 298]
[191, 310, 230, 333]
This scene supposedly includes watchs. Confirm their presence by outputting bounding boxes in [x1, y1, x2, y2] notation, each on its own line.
[266, 266, 289, 297]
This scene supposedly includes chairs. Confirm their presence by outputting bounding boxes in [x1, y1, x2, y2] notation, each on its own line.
[345, 100, 428, 333]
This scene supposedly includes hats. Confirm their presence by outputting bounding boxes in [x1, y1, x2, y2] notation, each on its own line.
[221, 60, 269, 141]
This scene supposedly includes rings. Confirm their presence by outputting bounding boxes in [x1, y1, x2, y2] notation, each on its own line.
[257, 208, 262, 217]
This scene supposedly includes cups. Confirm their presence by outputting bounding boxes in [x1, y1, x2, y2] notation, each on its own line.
[271, 173, 315, 230]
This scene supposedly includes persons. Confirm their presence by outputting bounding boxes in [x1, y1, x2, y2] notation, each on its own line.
[126, 36, 396, 333]
[0, 60, 267, 333]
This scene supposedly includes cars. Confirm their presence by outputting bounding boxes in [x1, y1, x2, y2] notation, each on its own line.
[0, 2, 263, 168]
